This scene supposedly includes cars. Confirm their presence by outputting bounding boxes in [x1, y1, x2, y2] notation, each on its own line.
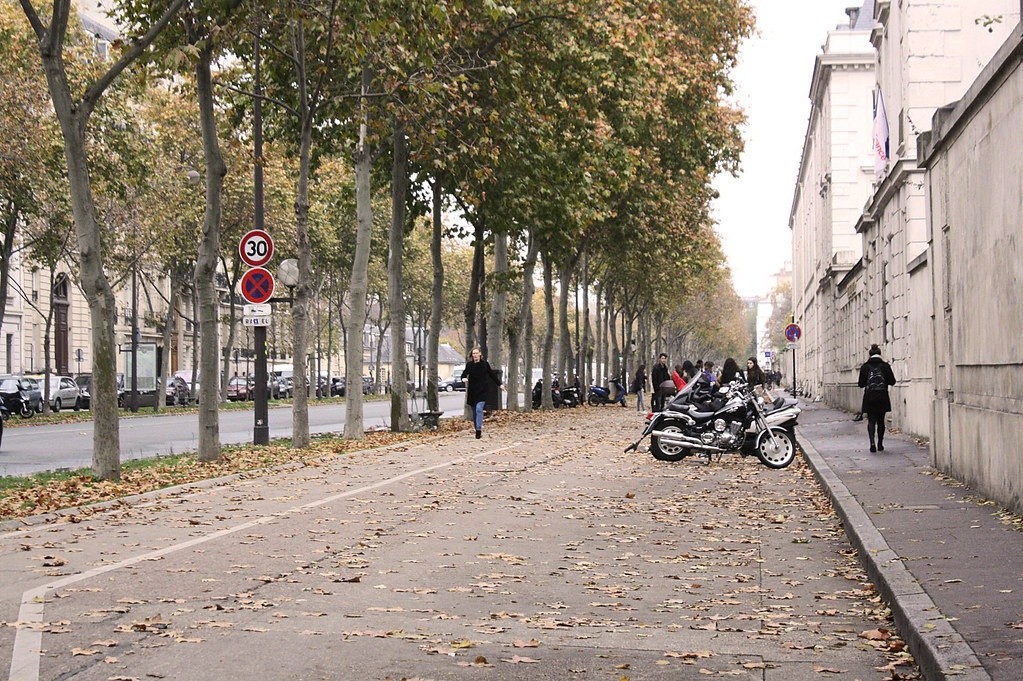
[0, 367, 468, 412]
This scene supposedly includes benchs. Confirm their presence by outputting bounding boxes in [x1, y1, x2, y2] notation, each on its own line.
[408, 410, 444, 428]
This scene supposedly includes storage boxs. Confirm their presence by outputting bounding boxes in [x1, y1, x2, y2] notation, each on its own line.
[659, 380, 675, 396]
[332, 377, 340, 385]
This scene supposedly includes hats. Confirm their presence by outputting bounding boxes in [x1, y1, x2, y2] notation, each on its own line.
[869, 344, 881, 356]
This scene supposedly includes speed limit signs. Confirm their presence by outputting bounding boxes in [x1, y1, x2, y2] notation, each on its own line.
[238, 229, 274, 267]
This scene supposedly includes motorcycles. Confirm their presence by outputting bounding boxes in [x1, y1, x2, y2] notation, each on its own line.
[532, 372, 579, 410]
[643, 368, 802, 469]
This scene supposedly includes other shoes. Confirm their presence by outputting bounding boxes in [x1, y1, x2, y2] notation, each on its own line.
[878, 447, 883, 451]
[642, 410, 648, 414]
[636, 411, 642, 414]
[870, 448, 876, 452]
[476, 430, 481, 439]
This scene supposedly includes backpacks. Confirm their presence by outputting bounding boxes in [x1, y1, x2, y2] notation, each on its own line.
[866, 363, 886, 392]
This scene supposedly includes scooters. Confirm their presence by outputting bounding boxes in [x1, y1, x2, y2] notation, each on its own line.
[0, 379, 36, 421]
[585, 372, 628, 407]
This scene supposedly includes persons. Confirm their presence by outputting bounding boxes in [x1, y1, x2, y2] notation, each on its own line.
[652, 353, 671, 412]
[461, 347, 505, 438]
[675, 358, 746, 389]
[746, 358, 782, 391]
[859, 344, 896, 453]
[636, 365, 647, 412]
